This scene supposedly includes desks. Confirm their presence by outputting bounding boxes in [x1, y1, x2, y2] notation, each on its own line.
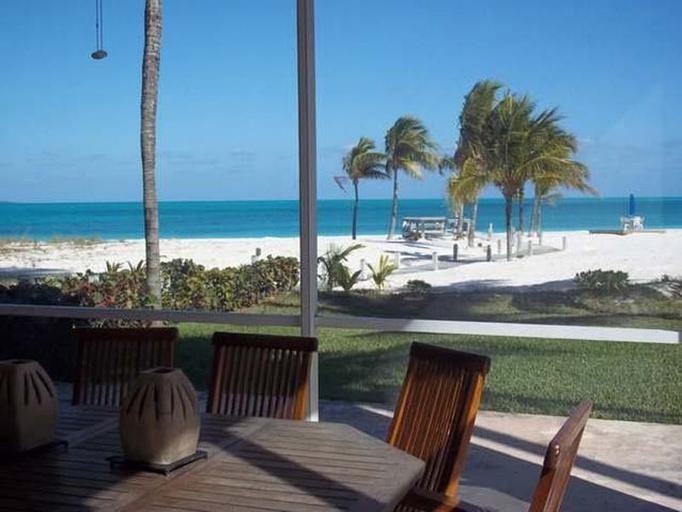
[0, 407, 426, 512]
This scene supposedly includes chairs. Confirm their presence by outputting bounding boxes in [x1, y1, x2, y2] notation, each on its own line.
[384, 341, 491, 497]
[72, 327, 177, 411]
[396, 399, 593, 511]
[207, 333, 317, 421]
